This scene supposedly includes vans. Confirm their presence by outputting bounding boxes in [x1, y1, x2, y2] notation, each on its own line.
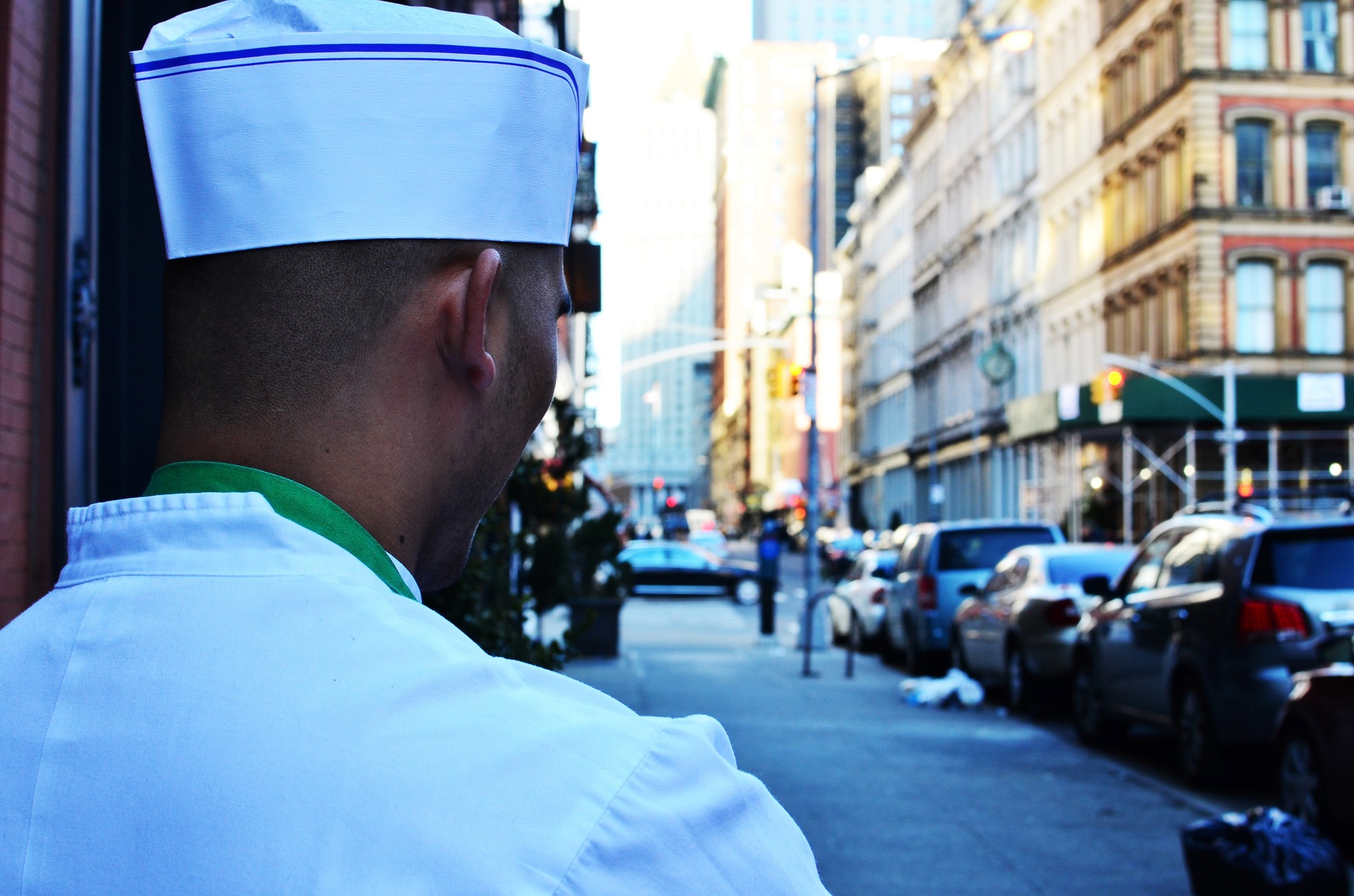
[871, 521, 1068, 679]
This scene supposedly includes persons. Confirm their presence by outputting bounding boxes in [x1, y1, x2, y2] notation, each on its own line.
[1, 1, 839, 892]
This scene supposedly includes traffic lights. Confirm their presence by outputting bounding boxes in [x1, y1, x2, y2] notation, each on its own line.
[777, 361, 804, 403]
[1089, 363, 1124, 406]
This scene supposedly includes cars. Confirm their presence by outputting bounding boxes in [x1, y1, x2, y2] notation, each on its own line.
[811, 520, 921, 654]
[616, 540, 764, 608]
[949, 542, 1138, 713]
[1276, 632, 1354, 845]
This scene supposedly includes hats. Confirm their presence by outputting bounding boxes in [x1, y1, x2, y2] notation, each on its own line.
[130, 0, 590, 260]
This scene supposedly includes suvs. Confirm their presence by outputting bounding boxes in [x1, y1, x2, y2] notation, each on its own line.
[1066, 492, 1354, 799]
